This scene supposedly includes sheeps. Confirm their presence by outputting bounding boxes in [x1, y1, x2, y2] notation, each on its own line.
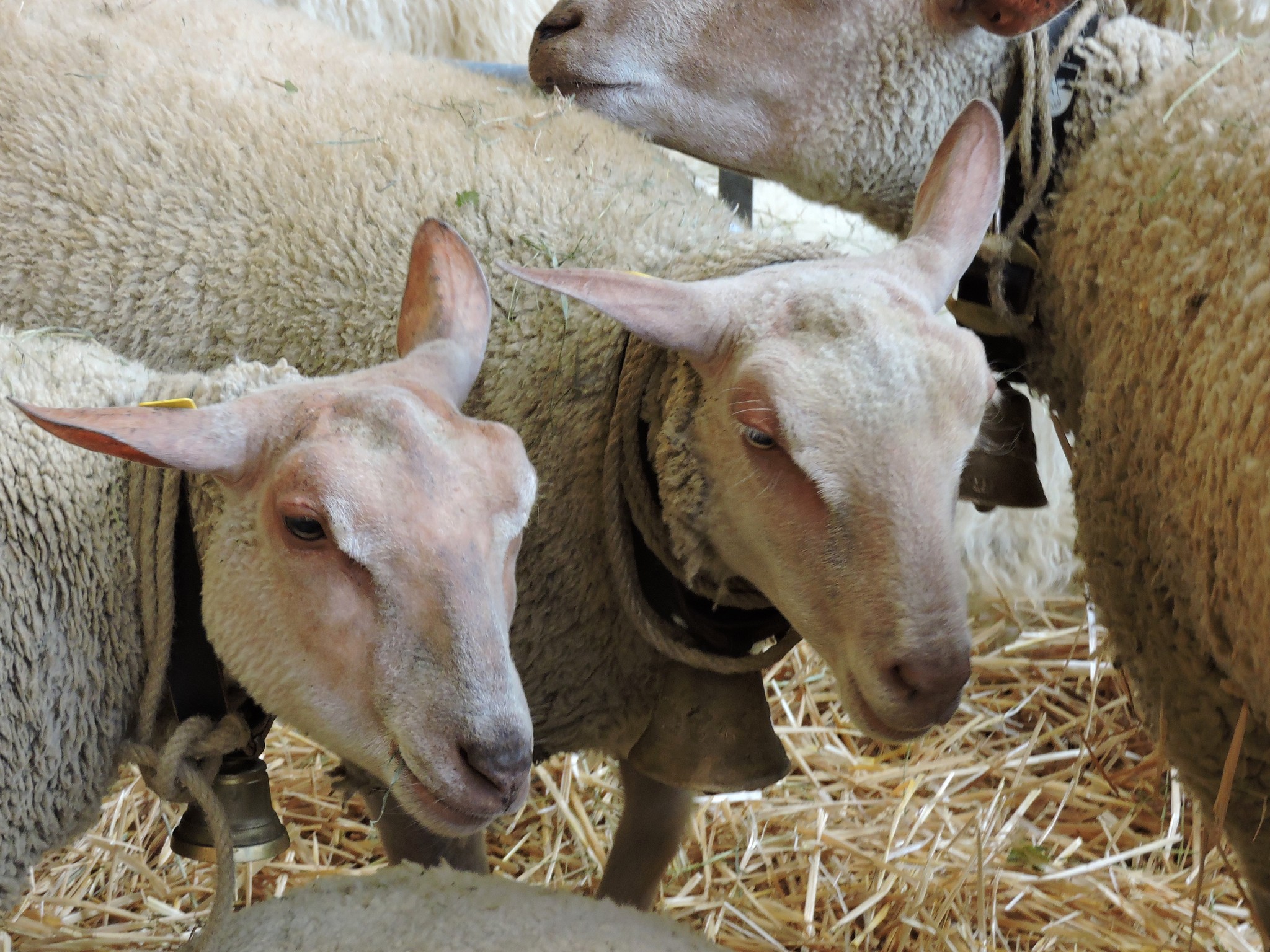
[180, 857, 730, 952]
[3, 0, 1005, 907]
[1, 213, 542, 929]
[521, 1, 1270, 952]
